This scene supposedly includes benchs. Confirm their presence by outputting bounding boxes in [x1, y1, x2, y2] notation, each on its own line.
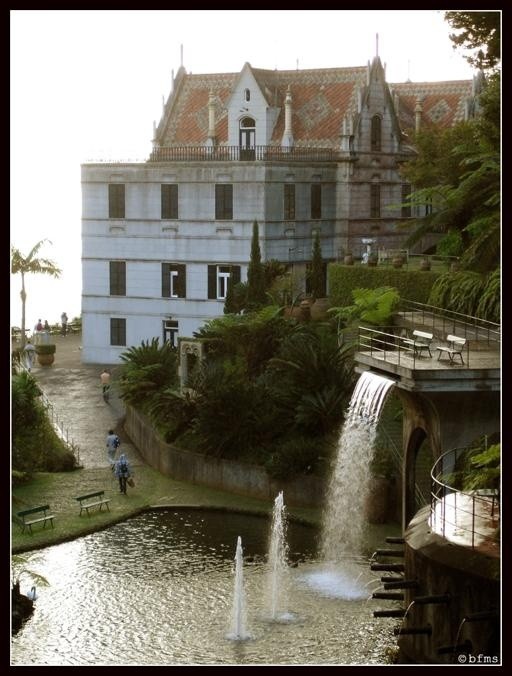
[17, 505, 56, 535]
[436, 334, 467, 365]
[403, 330, 433, 359]
[77, 491, 112, 518]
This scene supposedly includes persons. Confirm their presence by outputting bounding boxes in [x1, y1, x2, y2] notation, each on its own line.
[106, 429, 119, 470]
[61, 312, 69, 337]
[43, 320, 51, 342]
[101, 369, 110, 402]
[114, 455, 130, 494]
[34, 319, 43, 344]
[24, 341, 35, 372]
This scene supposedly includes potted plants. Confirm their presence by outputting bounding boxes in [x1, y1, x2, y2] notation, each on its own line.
[34, 344, 56, 367]
